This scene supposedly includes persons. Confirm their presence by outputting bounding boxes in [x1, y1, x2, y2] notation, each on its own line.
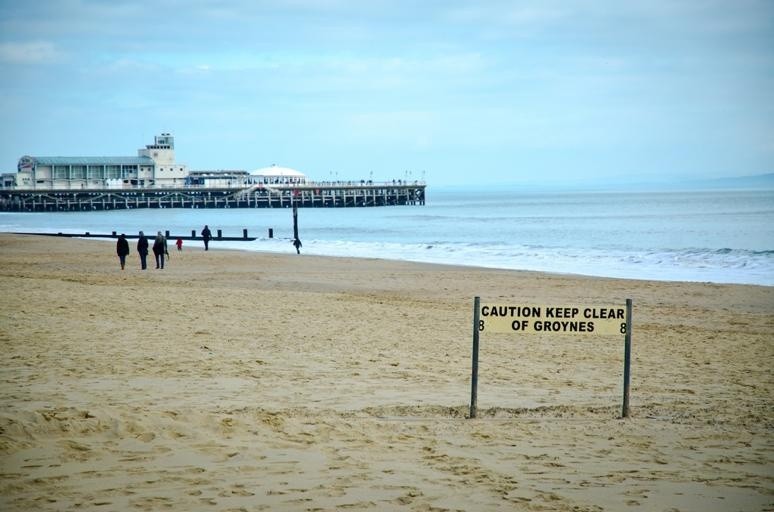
[175, 237, 183, 252]
[200, 225, 213, 252]
[116, 234, 129, 272]
[153, 231, 168, 270]
[136, 230, 149, 270]
